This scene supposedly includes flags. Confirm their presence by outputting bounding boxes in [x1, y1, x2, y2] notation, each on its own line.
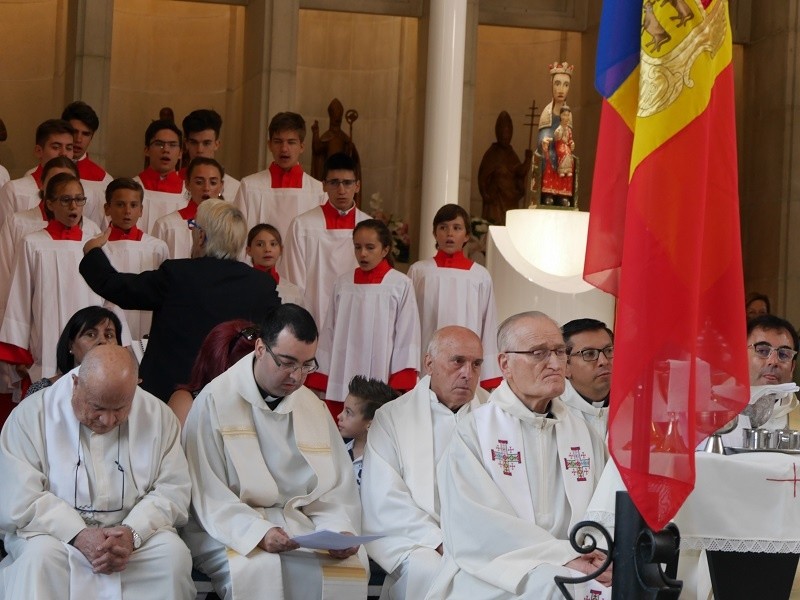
[581, 0, 751, 532]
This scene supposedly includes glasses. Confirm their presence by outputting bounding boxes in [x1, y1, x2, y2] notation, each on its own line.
[505, 341, 571, 364]
[571, 345, 615, 361]
[262, 339, 319, 374]
[228, 328, 263, 356]
[325, 177, 358, 189]
[747, 344, 799, 363]
[188, 219, 208, 241]
[52, 196, 87, 207]
[74, 458, 124, 513]
[149, 139, 182, 152]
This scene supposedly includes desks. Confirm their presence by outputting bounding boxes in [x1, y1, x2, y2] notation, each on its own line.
[580, 448, 800, 600]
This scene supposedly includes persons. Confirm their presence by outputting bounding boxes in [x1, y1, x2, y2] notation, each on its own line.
[0, 155, 102, 431]
[746, 292, 770, 322]
[477, 110, 532, 226]
[167, 320, 260, 425]
[0, 173, 115, 398]
[745, 313, 800, 385]
[310, 97, 361, 209]
[554, 318, 614, 439]
[537, 61, 576, 207]
[182, 303, 370, 600]
[79, 198, 281, 403]
[407, 204, 503, 392]
[304, 220, 421, 442]
[359, 326, 493, 600]
[436, 311, 614, 600]
[25, 306, 121, 396]
[336, 375, 400, 490]
[0, 346, 198, 600]
[0, 100, 375, 345]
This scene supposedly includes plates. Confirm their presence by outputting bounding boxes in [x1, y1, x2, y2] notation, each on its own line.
[723, 446, 799, 455]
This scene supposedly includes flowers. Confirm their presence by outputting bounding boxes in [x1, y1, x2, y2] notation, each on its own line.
[370, 192, 412, 262]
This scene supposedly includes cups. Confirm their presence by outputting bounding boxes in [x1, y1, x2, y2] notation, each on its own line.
[774, 429, 800, 450]
[743, 428, 771, 449]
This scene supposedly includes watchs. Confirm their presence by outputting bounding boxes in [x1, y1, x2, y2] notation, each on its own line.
[128, 526, 142, 551]
